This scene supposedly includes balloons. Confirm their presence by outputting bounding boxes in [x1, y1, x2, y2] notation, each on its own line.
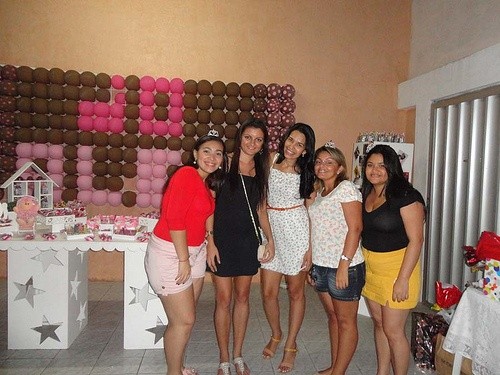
[138, 76, 183, 138]
[266, 83, 295, 113]
[78, 71, 140, 134]
[196, 109, 226, 138]
[266, 112, 295, 152]
[0, 64, 81, 202]
[182, 79, 197, 166]
[226, 110, 253, 153]
[76, 131, 182, 208]
[225, 82, 253, 112]
[197, 80, 226, 110]
[253, 84, 267, 121]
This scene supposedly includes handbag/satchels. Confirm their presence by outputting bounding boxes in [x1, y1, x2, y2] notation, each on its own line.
[257, 245, 270, 261]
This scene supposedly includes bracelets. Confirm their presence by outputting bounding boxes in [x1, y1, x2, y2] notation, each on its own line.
[177, 256, 190, 263]
[205, 230, 214, 240]
[340, 255, 352, 263]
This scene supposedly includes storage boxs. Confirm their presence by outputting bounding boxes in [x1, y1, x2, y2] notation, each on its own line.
[410, 312, 450, 372]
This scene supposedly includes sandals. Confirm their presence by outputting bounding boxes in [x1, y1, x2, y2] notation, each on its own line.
[277, 343, 297, 373]
[262, 331, 282, 359]
[218, 362, 232, 375]
[232, 356, 250, 375]
[181, 366, 197, 375]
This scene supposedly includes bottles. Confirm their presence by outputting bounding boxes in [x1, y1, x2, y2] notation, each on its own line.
[357, 132, 406, 143]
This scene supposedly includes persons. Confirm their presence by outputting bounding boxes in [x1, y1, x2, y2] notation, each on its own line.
[145, 129, 230, 375]
[356, 145, 428, 375]
[257, 122, 312, 373]
[298, 141, 368, 375]
[208, 118, 277, 375]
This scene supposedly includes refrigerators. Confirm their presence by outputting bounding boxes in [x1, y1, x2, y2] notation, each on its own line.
[352, 143, 414, 318]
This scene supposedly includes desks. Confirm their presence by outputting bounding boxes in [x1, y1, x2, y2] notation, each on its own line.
[442, 283, 500, 375]
[0, 202, 170, 350]
